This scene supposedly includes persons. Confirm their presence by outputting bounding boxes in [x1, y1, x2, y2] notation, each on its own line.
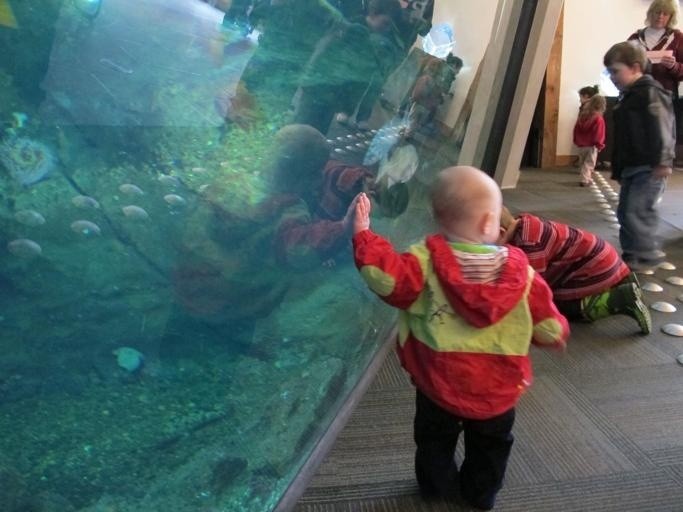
[159, 120, 378, 363]
[577, 85, 616, 170]
[492, 195, 651, 336]
[399, 52, 463, 143]
[623, 1, 682, 173]
[350, 165, 569, 511]
[602, 42, 677, 272]
[572, 93, 607, 187]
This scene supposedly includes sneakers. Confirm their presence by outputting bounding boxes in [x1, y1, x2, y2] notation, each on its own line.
[622, 252, 668, 266]
[580, 180, 592, 186]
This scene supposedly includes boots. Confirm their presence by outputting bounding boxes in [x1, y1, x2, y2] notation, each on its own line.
[581, 271, 651, 333]
